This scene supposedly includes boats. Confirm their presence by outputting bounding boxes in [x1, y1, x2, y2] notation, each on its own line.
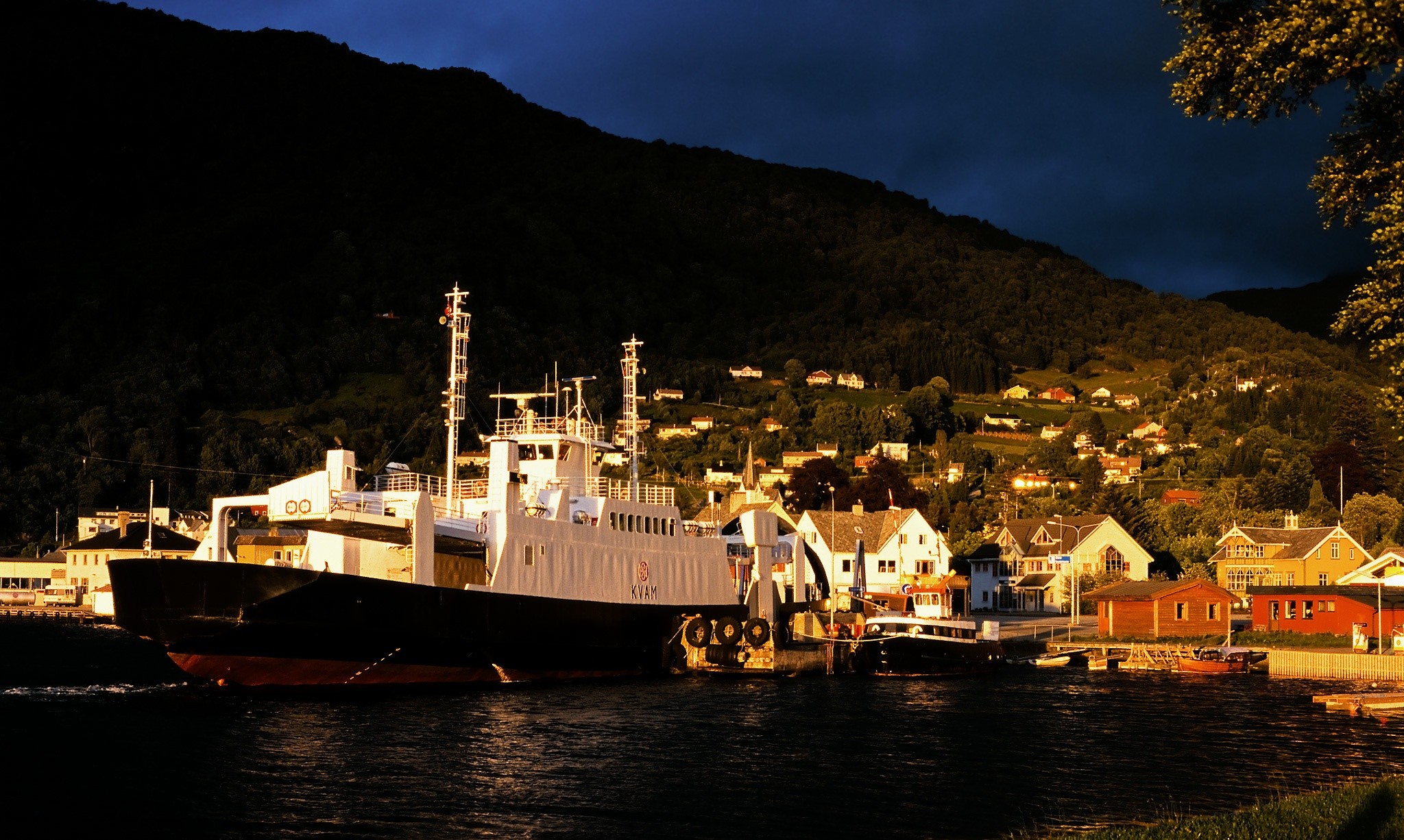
[107, 280, 791, 694]
